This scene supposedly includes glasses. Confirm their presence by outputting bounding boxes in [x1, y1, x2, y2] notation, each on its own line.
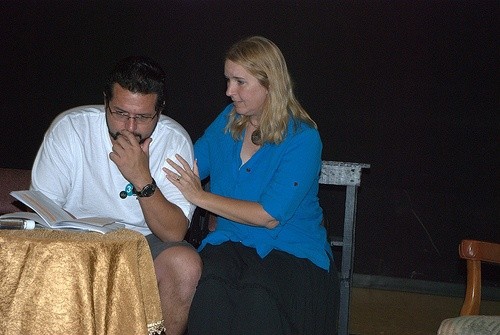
[108, 99, 160, 122]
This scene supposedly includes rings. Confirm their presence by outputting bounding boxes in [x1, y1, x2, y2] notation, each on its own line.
[176, 176, 181, 180]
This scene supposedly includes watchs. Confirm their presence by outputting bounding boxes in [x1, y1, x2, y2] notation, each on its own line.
[133, 178, 157, 197]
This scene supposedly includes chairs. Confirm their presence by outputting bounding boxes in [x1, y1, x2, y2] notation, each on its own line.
[204, 160, 371, 335]
[438, 239, 500, 335]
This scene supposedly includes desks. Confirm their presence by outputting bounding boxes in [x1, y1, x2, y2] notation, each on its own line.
[0, 228, 167, 335]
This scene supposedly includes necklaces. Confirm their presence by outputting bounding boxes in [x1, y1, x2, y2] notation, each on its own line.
[250, 122, 261, 145]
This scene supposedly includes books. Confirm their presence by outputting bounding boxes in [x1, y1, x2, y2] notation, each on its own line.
[0, 190, 125, 235]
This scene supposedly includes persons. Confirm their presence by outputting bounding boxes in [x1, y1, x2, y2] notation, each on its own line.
[162, 37, 339, 335]
[29, 43, 202, 335]
